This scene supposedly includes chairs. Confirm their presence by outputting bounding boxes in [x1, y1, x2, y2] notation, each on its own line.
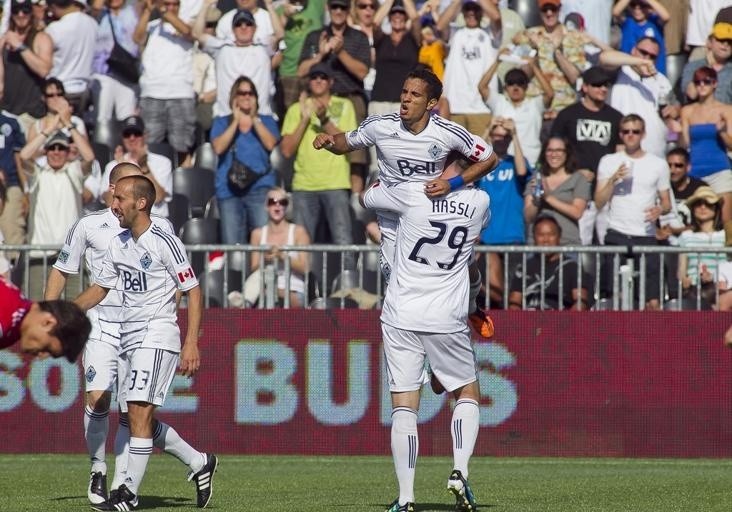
[363, 253, 379, 272]
[199, 269, 242, 307]
[307, 297, 358, 309]
[90, 142, 110, 175]
[372, 299, 385, 310]
[590, 297, 647, 311]
[331, 269, 386, 310]
[269, 144, 293, 184]
[665, 55, 690, 105]
[173, 168, 215, 217]
[309, 252, 341, 303]
[315, 204, 357, 244]
[350, 192, 377, 226]
[663, 298, 711, 310]
[167, 193, 192, 238]
[179, 217, 222, 279]
[580, 245, 607, 277]
[147, 142, 175, 170]
[509, 0, 544, 28]
[92, 120, 128, 161]
[204, 196, 220, 219]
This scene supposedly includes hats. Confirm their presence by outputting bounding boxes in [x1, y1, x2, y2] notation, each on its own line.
[582, 66, 613, 84]
[505, 68, 528, 82]
[327, 0, 346, 7]
[707, 21, 732, 41]
[46, 131, 71, 148]
[537, 1, 561, 8]
[387, 1, 406, 13]
[309, 63, 333, 76]
[232, 9, 255, 25]
[121, 116, 145, 133]
[686, 186, 725, 206]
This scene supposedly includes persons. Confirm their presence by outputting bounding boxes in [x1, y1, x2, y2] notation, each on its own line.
[213, 0, 286, 128]
[509, 216, 595, 310]
[680, 22, 732, 105]
[681, 66, 732, 251]
[359, 149, 492, 512]
[33, 130, 104, 225]
[35, 161, 183, 506]
[209, 74, 282, 273]
[541, 65, 623, 201]
[478, 47, 555, 173]
[0, 0, 55, 143]
[25, 78, 89, 151]
[279, 62, 356, 272]
[367, 1, 423, 185]
[595, 114, 673, 311]
[717, 286, 732, 312]
[412, 0, 447, 80]
[87, 0, 145, 149]
[510, 1, 586, 119]
[274, 0, 329, 110]
[598, 37, 681, 163]
[131, 0, 199, 171]
[298, 0, 371, 219]
[351, 1, 384, 101]
[85, 0, 110, 26]
[17, 97, 97, 302]
[190, 1, 286, 124]
[657, 148, 712, 246]
[70, 175, 220, 511]
[226, 186, 311, 309]
[45, 0, 102, 119]
[0, 82, 28, 217]
[188, 7, 223, 35]
[523, 136, 592, 275]
[178, 41, 215, 162]
[436, 0, 501, 115]
[0, 273, 91, 364]
[312, 62, 501, 395]
[472, 114, 534, 309]
[677, 184, 731, 313]
[612, 0, 670, 78]
[1, 174, 15, 279]
[98, 116, 177, 220]
[564, 11, 611, 52]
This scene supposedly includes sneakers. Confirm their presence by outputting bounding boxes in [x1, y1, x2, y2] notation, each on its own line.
[431, 372, 445, 396]
[383, 499, 413, 512]
[188, 453, 220, 508]
[468, 307, 494, 338]
[88, 470, 109, 504]
[90, 484, 140, 512]
[448, 469, 476, 512]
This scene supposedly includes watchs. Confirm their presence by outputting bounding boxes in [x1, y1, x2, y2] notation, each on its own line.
[254, 117, 262, 129]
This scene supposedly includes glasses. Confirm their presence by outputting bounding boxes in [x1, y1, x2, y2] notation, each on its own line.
[267, 198, 288, 207]
[124, 132, 141, 139]
[11, 6, 31, 14]
[694, 78, 713, 87]
[49, 144, 67, 151]
[359, 4, 377, 9]
[544, 148, 567, 155]
[46, 93, 64, 98]
[236, 91, 254, 97]
[623, 128, 642, 136]
[635, 47, 658, 61]
[667, 163, 688, 170]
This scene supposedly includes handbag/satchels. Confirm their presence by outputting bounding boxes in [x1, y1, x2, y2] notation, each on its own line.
[105, 9, 141, 85]
[227, 146, 272, 198]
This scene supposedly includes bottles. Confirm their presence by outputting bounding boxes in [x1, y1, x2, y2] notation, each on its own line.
[533, 162, 543, 198]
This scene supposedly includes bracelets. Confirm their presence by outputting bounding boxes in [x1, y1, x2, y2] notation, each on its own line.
[42, 131, 51, 141]
[717, 123, 728, 135]
[66, 124, 77, 132]
[161, 6, 169, 17]
[320, 117, 329, 126]
[143, 169, 150, 176]
